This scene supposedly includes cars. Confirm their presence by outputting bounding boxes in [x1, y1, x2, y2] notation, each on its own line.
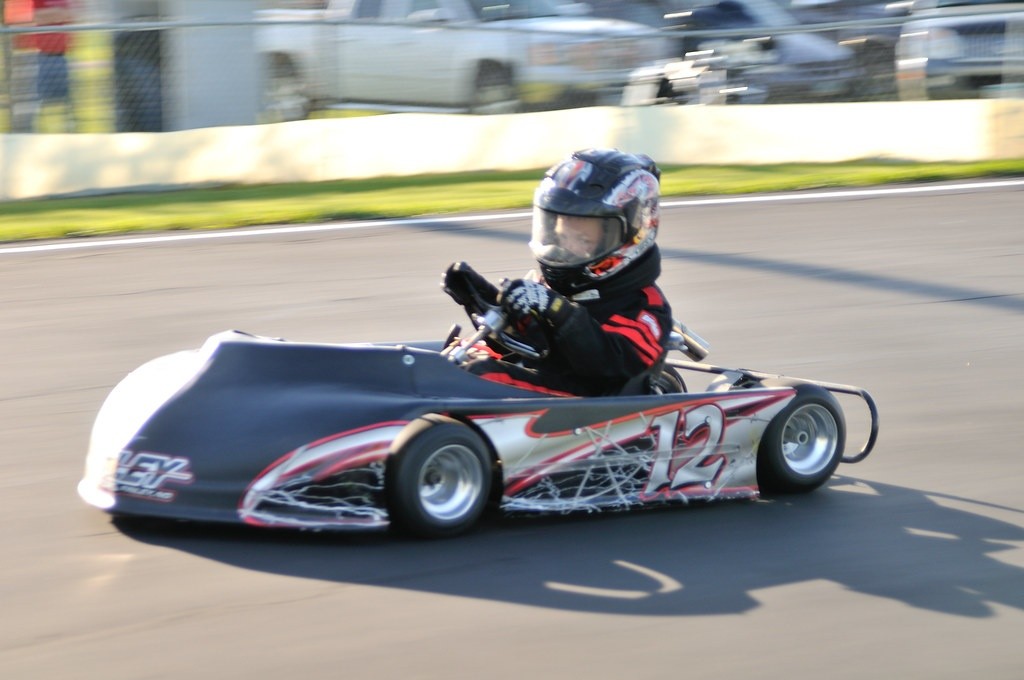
[572, 2, 1024, 100]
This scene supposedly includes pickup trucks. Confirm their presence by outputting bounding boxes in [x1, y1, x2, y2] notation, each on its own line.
[255, 1, 672, 115]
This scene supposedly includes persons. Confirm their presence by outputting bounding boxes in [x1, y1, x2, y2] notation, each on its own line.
[439, 145, 684, 404]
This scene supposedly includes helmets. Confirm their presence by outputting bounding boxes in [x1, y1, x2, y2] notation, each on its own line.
[529, 149, 667, 301]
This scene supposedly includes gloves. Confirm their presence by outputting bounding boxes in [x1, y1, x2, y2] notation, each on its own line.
[500, 279, 573, 332]
